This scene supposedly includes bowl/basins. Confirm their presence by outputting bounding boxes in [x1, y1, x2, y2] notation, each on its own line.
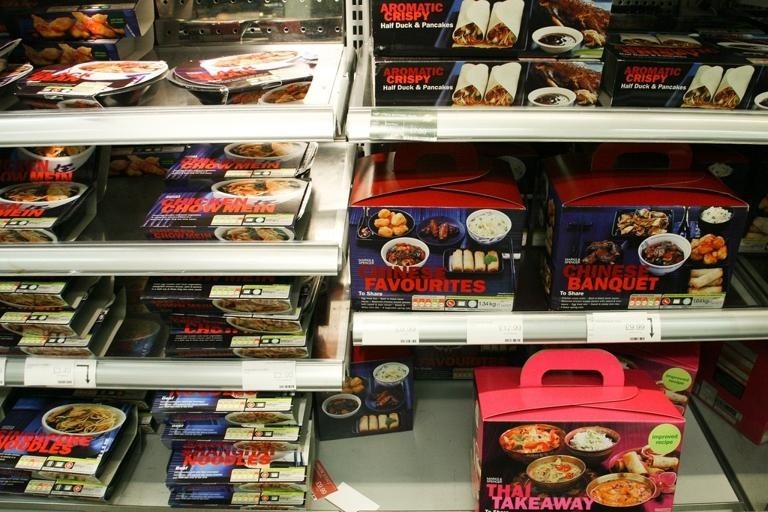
[216, 52, 313, 108]
[380, 235, 431, 269]
[0, 146, 97, 242]
[56, 61, 166, 107]
[698, 207, 736, 230]
[323, 392, 360, 419]
[523, 87, 579, 108]
[372, 362, 410, 386]
[499, 423, 682, 508]
[41, 403, 126, 441]
[367, 207, 416, 238]
[636, 232, 693, 273]
[212, 141, 307, 247]
[463, 209, 513, 244]
[531, 25, 588, 55]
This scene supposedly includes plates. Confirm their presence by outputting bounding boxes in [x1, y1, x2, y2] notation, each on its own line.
[0, 292, 95, 356]
[226, 414, 304, 511]
[412, 216, 467, 248]
[117, 318, 162, 343]
[433, 0, 534, 53]
[350, 412, 403, 432]
[366, 387, 406, 410]
[340, 374, 371, 399]
[611, 208, 674, 236]
[431, 62, 533, 106]
[211, 299, 309, 361]
[441, 247, 506, 277]
[615, 28, 768, 111]
[580, 240, 628, 270]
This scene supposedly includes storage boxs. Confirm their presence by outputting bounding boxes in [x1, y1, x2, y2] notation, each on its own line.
[314, 346, 701, 511]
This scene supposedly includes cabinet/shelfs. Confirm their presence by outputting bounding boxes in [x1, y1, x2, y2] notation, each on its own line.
[1, 1, 356, 510]
[345, 1, 767, 345]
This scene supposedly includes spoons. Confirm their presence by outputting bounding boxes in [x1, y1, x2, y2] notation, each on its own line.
[677, 206, 692, 238]
[358, 206, 371, 239]
